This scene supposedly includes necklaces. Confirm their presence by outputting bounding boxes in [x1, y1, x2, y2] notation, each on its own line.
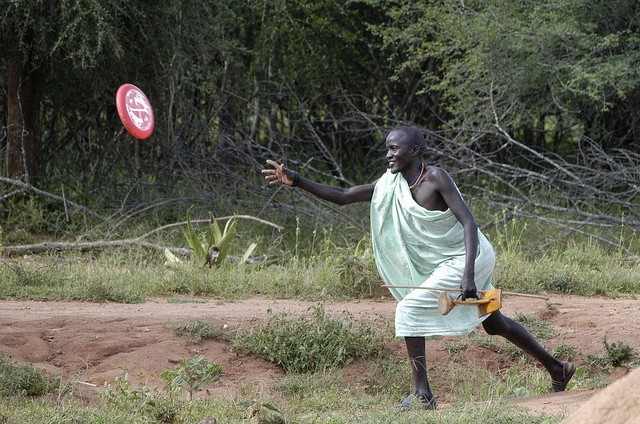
[388, 159, 426, 190]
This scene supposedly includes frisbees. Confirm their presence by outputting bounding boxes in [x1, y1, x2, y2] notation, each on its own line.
[115, 83, 155, 140]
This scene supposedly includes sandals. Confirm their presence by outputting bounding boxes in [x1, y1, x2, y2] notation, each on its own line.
[551, 361, 576, 392]
[395, 394, 436, 411]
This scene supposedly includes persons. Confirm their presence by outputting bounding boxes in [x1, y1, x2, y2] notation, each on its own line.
[260, 126, 578, 413]
